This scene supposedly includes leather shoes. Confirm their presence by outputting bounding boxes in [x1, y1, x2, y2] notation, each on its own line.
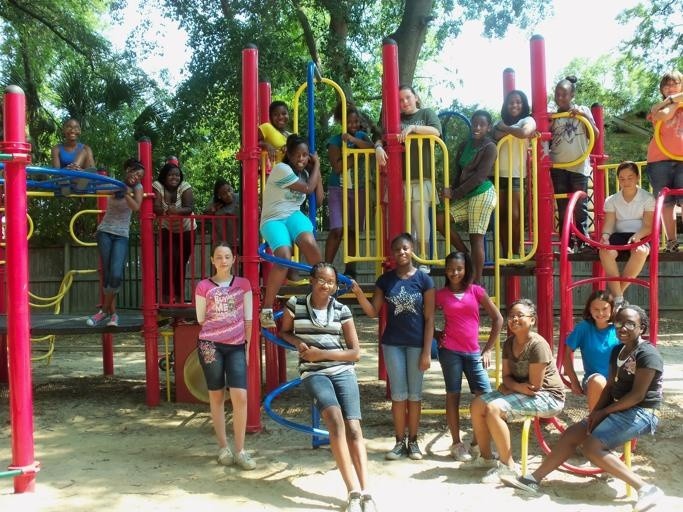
[288, 278, 310, 286]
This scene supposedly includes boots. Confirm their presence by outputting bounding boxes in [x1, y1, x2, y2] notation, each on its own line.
[408, 435, 423, 460]
[386, 434, 406, 460]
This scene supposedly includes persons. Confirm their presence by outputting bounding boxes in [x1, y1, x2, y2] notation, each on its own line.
[152, 162, 197, 329]
[436, 90, 536, 285]
[325, 85, 443, 277]
[460, 290, 665, 512]
[350, 232, 504, 460]
[258, 101, 324, 328]
[89, 155, 146, 330]
[279, 262, 379, 512]
[51, 117, 96, 198]
[547, 71, 682, 309]
[194, 239, 264, 470]
[202, 179, 243, 239]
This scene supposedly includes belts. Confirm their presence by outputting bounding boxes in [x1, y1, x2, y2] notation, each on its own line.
[614, 399, 660, 419]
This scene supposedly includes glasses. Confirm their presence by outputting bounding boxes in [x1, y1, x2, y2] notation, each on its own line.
[614, 321, 640, 330]
[312, 275, 334, 285]
[508, 313, 532, 319]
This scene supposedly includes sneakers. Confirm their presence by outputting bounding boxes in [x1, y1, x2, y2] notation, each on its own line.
[218, 448, 234, 466]
[450, 442, 539, 494]
[360, 495, 376, 512]
[235, 452, 256, 470]
[86, 310, 108, 326]
[634, 484, 664, 512]
[107, 312, 118, 326]
[559, 242, 575, 253]
[611, 299, 629, 320]
[666, 240, 679, 252]
[346, 493, 362, 512]
[577, 244, 592, 253]
[260, 311, 276, 328]
